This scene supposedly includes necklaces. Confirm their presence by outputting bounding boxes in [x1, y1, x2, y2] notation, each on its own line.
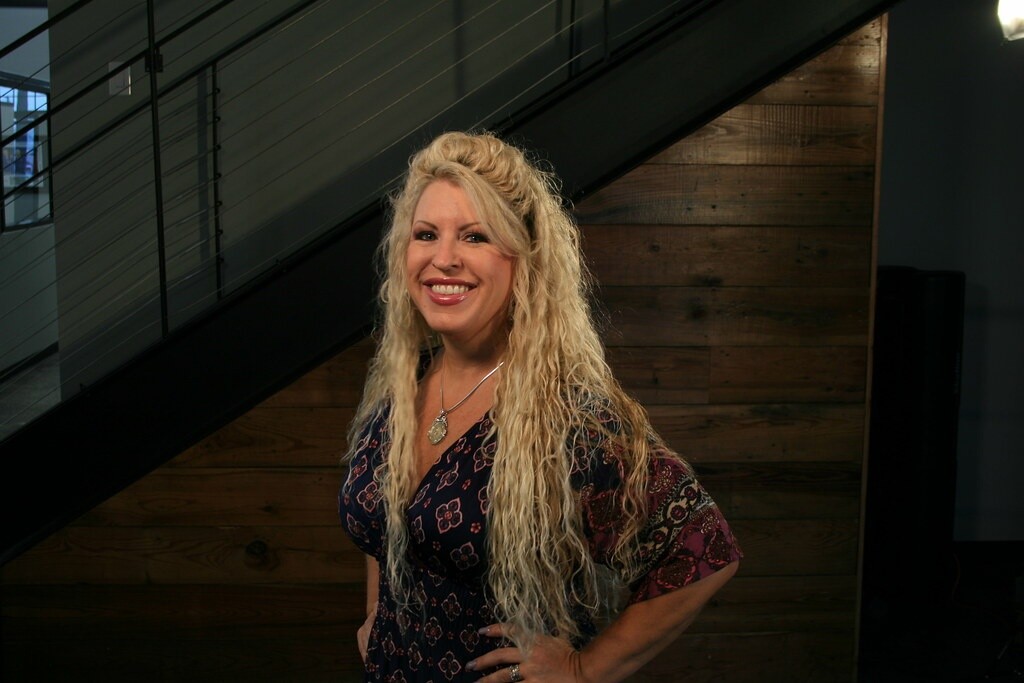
[427, 349, 504, 445]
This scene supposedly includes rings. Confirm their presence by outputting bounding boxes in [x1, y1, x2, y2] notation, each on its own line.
[510, 664, 522, 681]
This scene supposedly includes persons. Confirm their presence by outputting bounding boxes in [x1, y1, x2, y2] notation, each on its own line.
[339, 131, 743, 683]
